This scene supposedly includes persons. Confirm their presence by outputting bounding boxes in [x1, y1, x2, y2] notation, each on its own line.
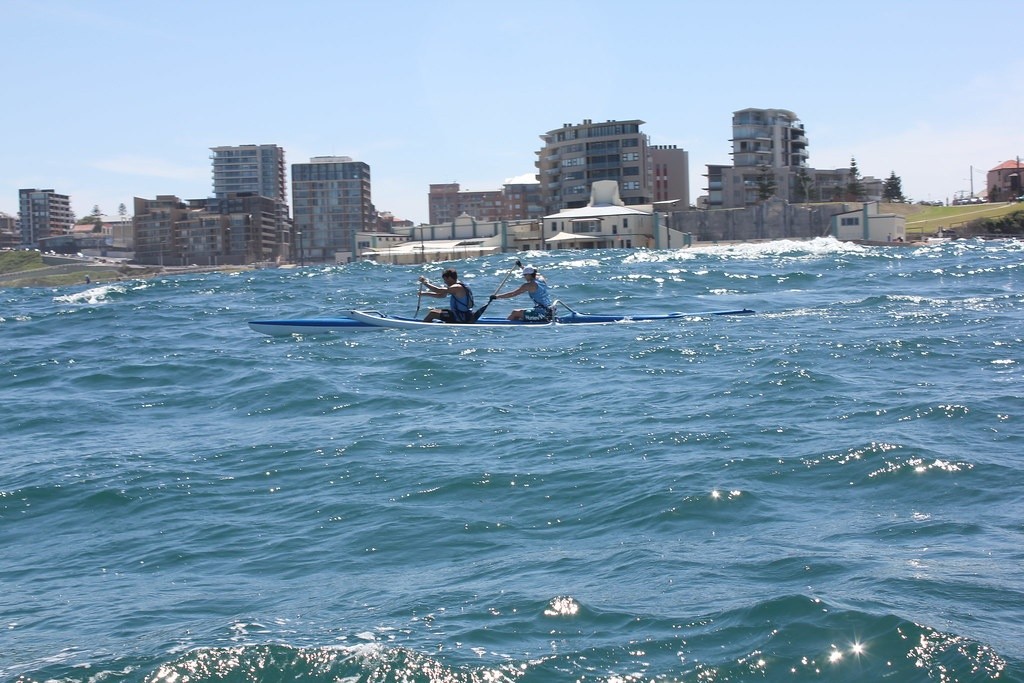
[489, 259, 554, 322]
[418, 267, 474, 324]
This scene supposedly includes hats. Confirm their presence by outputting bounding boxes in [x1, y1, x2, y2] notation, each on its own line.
[522, 267, 535, 275]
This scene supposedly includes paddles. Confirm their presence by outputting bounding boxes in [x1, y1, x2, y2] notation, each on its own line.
[473, 259, 518, 321]
[414, 282, 423, 318]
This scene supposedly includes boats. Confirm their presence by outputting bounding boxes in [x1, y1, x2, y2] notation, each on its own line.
[247, 309, 753, 336]
